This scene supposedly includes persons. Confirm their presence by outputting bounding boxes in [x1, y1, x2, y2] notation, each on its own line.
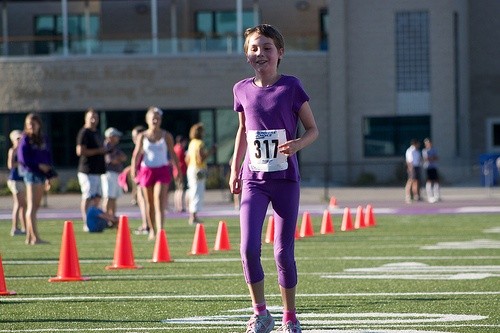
[404, 139, 424, 202]
[102, 127, 126, 214]
[422, 138, 443, 203]
[172, 134, 188, 212]
[75, 109, 112, 231]
[123, 125, 149, 234]
[6, 113, 53, 245]
[228, 24, 319, 333]
[184, 122, 218, 223]
[131, 107, 181, 240]
[86, 192, 119, 233]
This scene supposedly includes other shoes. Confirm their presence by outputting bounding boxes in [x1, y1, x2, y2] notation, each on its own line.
[10, 229, 26, 236]
[149, 231, 155, 240]
[135, 227, 149, 235]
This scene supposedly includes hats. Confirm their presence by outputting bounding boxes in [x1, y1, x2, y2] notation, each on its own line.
[104, 128, 122, 138]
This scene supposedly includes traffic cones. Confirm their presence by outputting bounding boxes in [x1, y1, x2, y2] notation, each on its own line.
[48, 220, 91, 281]
[190, 223, 209, 255]
[213, 220, 230, 251]
[328, 195, 337, 211]
[264, 216, 275, 242]
[300, 211, 313, 236]
[341, 203, 377, 232]
[106, 215, 143, 269]
[0, 255, 15, 295]
[320, 210, 335, 235]
[150, 230, 174, 263]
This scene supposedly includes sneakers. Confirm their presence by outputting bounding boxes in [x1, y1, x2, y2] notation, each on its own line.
[276, 321, 302, 333]
[246, 310, 275, 333]
